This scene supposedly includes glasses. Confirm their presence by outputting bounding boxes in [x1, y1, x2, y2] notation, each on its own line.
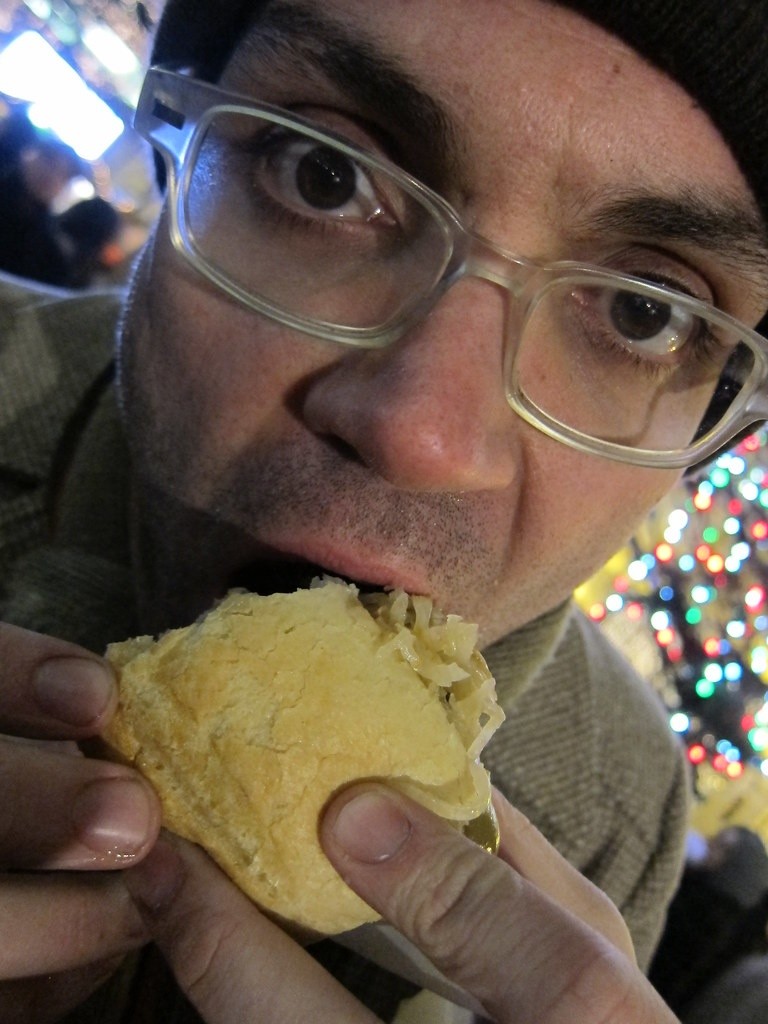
[133, 73, 767, 469]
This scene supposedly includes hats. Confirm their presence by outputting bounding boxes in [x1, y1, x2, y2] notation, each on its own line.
[148, 0, 768, 478]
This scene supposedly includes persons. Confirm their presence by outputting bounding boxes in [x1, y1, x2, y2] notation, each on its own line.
[0, 1, 768, 1024]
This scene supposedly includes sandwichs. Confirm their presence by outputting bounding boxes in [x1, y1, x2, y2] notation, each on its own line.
[78, 575, 505, 954]
